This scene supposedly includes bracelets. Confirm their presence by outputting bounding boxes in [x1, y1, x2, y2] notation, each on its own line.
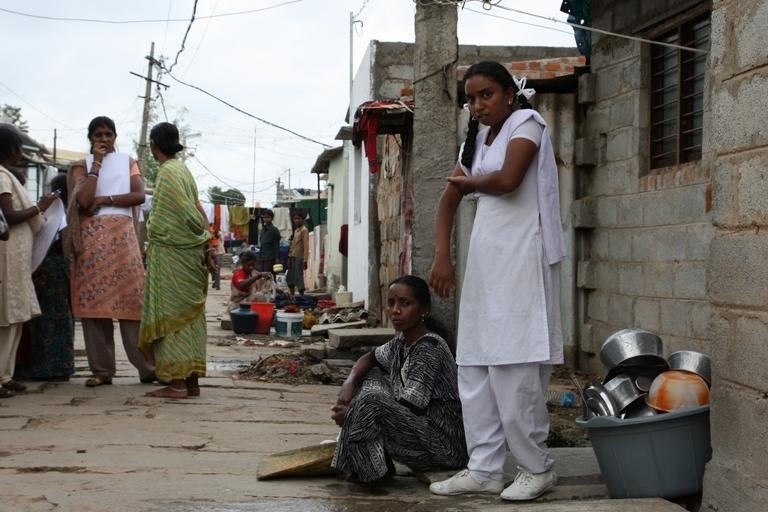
[207, 247, 216, 253]
[34, 203, 41, 215]
[92, 161, 103, 168]
[109, 196, 115, 206]
[88, 172, 98, 178]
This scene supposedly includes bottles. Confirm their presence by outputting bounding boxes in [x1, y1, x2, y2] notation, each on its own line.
[544, 390, 583, 408]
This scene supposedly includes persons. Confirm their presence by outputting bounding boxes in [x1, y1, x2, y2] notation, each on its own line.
[229, 251, 272, 310]
[66, 116, 158, 387]
[136, 122, 220, 399]
[427, 60, 566, 503]
[331, 275, 468, 486]
[1, 121, 61, 397]
[284, 210, 309, 295]
[13, 175, 76, 381]
[258, 210, 282, 284]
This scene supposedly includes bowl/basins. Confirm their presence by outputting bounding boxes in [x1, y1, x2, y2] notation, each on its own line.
[644, 371, 710, 413]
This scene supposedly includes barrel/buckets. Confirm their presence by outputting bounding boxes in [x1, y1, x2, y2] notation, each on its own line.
[574, 403, 710, 498]
[250, 303, 274, 333]
[276, 310, 305, 337]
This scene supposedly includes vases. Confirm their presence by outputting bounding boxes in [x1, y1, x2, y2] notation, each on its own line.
[230, 303, 258, 334]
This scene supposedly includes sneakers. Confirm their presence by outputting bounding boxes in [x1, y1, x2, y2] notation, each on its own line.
[428, 469, 505, 495]
[501, 464, 558, 500]
[86, 376, 112, 386]
[140, 369, 159, 383]
[0, 380, 26, 397]
